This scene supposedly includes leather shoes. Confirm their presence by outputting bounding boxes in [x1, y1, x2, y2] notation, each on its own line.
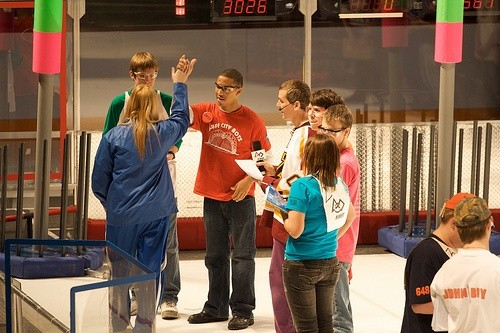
[228, 313, 254, 329]
[188, 309, 228, 323]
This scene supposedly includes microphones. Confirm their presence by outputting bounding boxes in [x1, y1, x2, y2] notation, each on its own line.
[254, 140, 265, 174]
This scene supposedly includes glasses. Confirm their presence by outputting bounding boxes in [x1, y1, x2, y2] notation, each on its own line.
[134, 72, 157, 79]
[278, 101, 297, 112]
[214, 82, 240, 94]
[318, 125, 348, 135]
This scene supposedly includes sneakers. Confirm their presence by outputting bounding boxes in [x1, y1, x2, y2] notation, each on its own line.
[160, 301, 178, 318]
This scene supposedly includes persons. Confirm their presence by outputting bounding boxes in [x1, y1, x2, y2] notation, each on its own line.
[308, 88, 344, 131]
[317, 105, 360, 333]
[282, 134, 355, 333]
[429, 197, 500, 333]
[400, 193, 474, 333]
[256, 80, 317, 333]
[91, 64, 190, 333]
[175, 54, 273, 330]
[101, 51, 183, 319]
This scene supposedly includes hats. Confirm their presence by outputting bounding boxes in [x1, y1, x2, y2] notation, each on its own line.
[445, 192, 476, 209]
[454, 197, 491, 227]
